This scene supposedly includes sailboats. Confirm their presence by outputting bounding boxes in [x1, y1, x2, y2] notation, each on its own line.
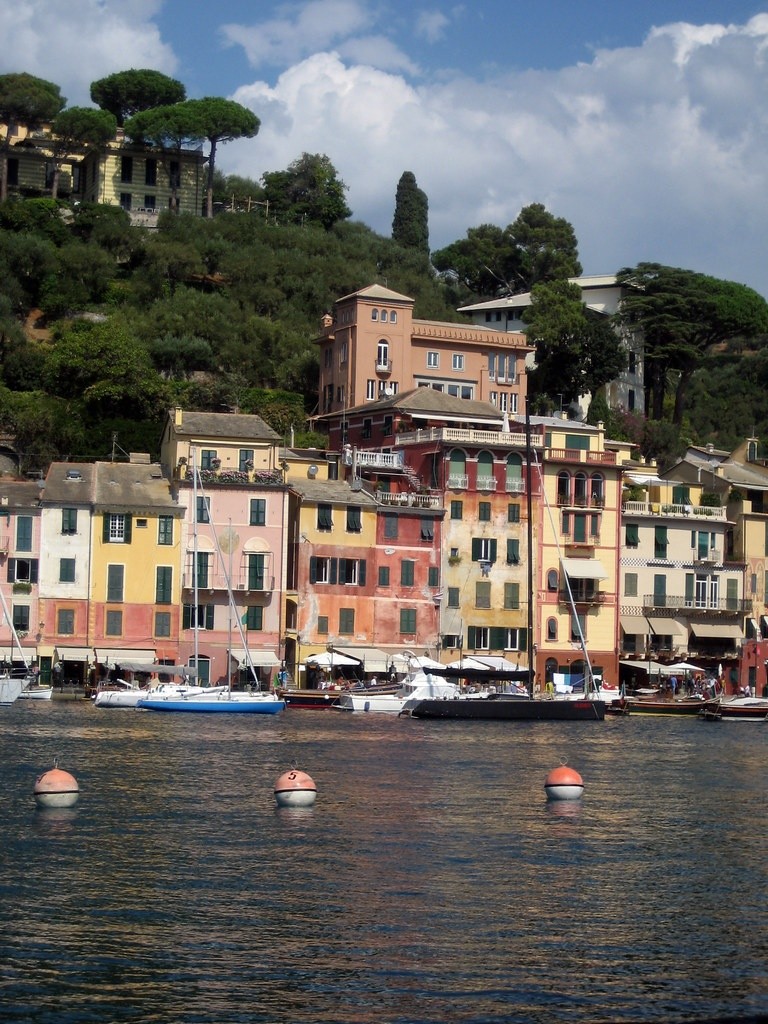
[0, 588, 34, 706]
[137, 446, 290, 714]
[399, 395, 605, 721]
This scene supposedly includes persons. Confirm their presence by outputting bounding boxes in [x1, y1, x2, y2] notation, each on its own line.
[337, 675, 344, 685]
[667, 674, 724, 697]
[32, 663, 42, 687]
[739, 683, 751, 697]
[390, 671, 396, 682]
[371, 675, 378, 686]
[179, 664, 189, 687]
[308, 668, 325, 689]
[278, 668, 287, 689]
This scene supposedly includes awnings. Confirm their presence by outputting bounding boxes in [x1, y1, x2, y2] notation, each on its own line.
[619, 615, 654, 635]
[95, 648, 157, 665]
[57, 648, 96, 662]
[226, 649, 286, 671]
[333, 646, 392, 672]
[560, 558, 609, 580]
[690, 622, 744, 638]
[0, 647, 36, 661]
[392, 654, 447, 673]
[648, 618, 682, 636]
[619, 660, 684, 675]
[466, 656, 529, 672]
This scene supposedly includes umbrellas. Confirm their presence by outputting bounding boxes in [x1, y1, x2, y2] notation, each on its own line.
[666, 662, 705, 686]
[446, 658, 490, 670]
[303, 652, 360, 685]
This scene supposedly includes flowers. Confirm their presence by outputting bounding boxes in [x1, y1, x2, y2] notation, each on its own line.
[244, 459, 255, 467]
[178, 456, 187, 464]
[185, 469, 283, 484]
[210, 458, 221, 463]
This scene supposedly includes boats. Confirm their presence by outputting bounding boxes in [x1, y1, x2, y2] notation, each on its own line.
[8, 632, 53, 699]
[275, 682, 408, 710]
[612, 697, 713, 717]
[422, 653, 535, 700]
[700, 694, 768, 721]
[90, 680, 230, 707]
[341, 690, 409, 714]
